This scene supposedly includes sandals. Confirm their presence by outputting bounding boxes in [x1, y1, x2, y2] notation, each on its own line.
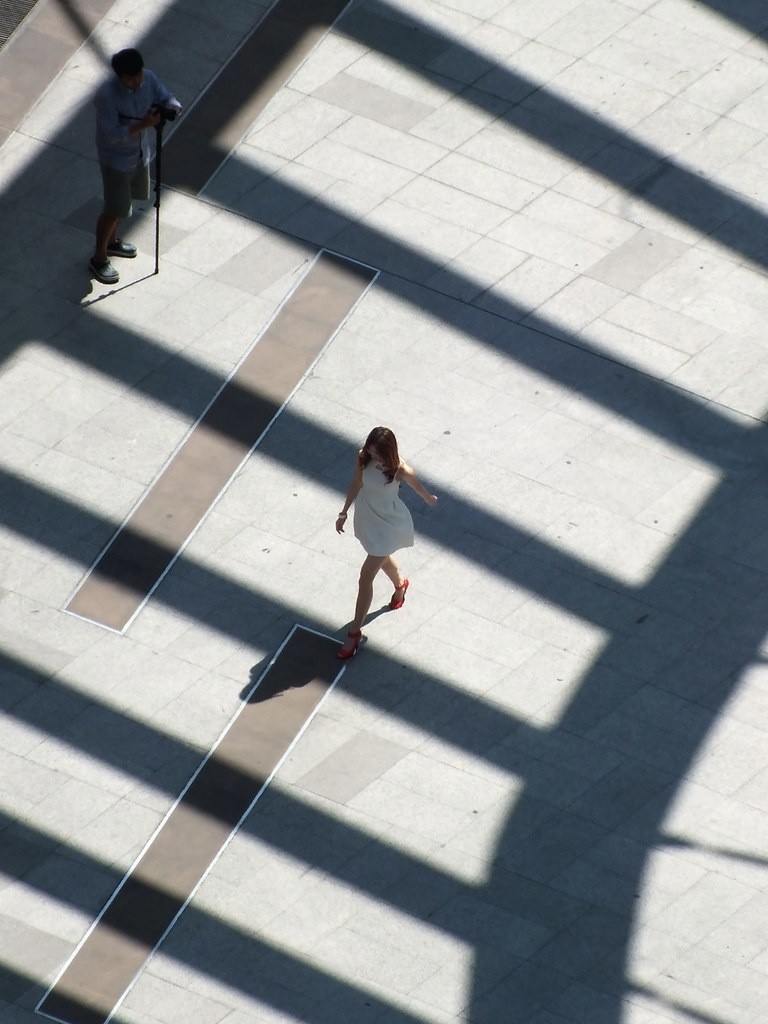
[97, 238, 137, 258]
[88, 257, 120, 283]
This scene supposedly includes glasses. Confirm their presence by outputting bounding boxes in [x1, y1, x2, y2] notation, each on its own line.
[366, 449, 379, 455]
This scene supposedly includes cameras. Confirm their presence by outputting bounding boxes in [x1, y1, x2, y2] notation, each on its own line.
[152, 103, 177, 130]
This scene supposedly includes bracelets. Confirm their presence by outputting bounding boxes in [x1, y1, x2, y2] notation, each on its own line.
[339, 513, 348, 520]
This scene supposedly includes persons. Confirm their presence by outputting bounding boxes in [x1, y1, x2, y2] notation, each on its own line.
[335, 426, 438, 659]
[88, 48, 184, 284]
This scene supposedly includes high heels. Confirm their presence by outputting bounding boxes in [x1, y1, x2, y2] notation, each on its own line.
[389, 579, 409, 609]
[336, 630, 363, 659]
